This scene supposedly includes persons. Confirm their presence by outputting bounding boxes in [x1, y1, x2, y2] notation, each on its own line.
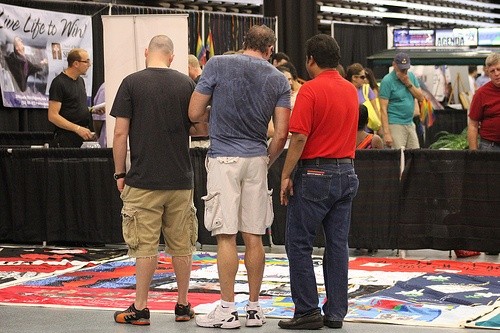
[379, 52, 425, 149]
[414, 98, 424, 147]
[188, 24, 292, 328]
[469, 66, 477, 94]
[188, 54, 211, 141]
[48, 48, 106, 148]
[467, 51, 500, 151]
[93, 84, 108, 148]
[355, 105, 383, 149]
[338, 63, 383, 136]
[266, 52, 304, 147]
[477, 62, 492, 88]
[441, 82, 456, 104]
[4, 37, 43, 92]
[109, 35, 196, 325]
[277, 34, 359, 329]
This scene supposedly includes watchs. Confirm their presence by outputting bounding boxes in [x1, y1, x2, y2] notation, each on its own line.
[114, 173, 126, 180]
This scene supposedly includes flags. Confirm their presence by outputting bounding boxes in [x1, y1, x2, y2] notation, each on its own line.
[196, 30, 214, 65]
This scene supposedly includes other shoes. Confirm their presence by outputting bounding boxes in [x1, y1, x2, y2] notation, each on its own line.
[323, 315, 342, 329]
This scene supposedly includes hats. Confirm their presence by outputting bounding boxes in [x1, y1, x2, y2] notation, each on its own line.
[395, 53, 410, 69]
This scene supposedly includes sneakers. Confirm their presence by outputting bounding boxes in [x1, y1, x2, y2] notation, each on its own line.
[195, 304, 241, 329]
[174, 302, 194, 321]
[114, 304, 149, 325]
[245, 305, 266, 327]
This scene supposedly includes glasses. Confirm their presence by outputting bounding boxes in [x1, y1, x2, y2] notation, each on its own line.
[78, 59, 90, 63]
[355, 76, 365, 79]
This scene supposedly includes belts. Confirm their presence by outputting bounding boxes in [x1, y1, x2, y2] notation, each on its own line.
[299, 158, 352, 166]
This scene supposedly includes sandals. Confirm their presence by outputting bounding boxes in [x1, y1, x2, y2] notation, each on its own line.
[278, 313, 324, 329]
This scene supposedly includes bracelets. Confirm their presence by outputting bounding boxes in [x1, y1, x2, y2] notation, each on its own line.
[76, 126, 80, 133]
[407, 84, 412, 88]
